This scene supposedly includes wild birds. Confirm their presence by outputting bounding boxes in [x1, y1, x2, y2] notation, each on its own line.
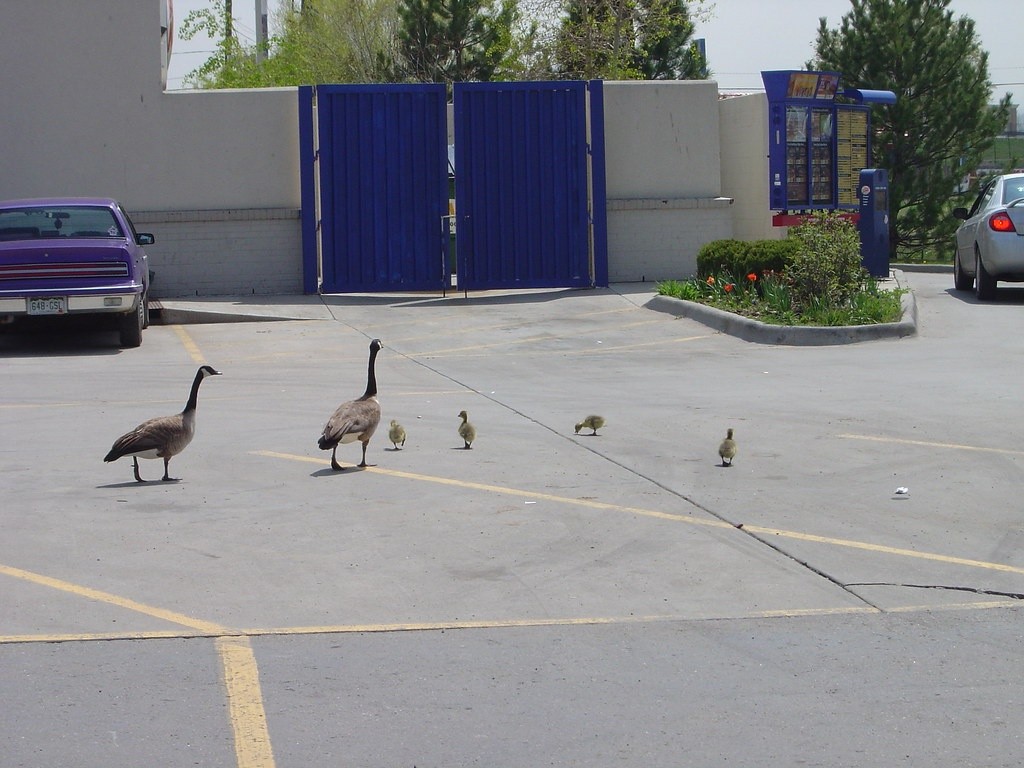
[573, 415, 606, 436]
[458, 410, 477, 449]
[316, 339, 406, 471]
[103, 366, 223, 483]
[717, 428, 736, 467]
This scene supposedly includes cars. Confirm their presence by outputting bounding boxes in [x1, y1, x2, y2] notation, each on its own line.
[950, 173, 1024, 300]
[0, 198, 155, 346]
